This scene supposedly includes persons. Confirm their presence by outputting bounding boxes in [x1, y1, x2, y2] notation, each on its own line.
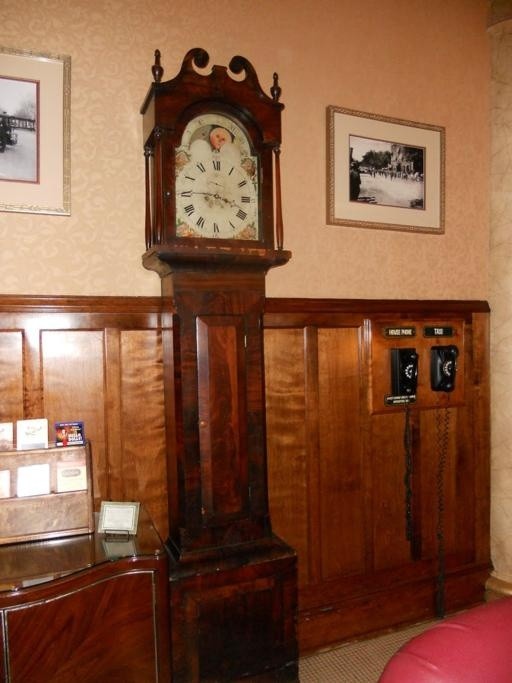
[349, 162, 361, 200]
[367, 164, 408, 180]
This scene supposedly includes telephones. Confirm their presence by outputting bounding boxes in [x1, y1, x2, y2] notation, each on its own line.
[391, 347, 418, 396]
[430, 344, 459, 392]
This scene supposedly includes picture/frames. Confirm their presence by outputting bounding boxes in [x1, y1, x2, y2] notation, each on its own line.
[326, 105, 447, 235]
[0, 46, 74, 216]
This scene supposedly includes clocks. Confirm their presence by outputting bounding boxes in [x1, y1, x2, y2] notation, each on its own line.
[138, 47, 302, 683]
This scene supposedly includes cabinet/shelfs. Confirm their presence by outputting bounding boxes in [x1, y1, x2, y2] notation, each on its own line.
[0, 501, 174, 683]
